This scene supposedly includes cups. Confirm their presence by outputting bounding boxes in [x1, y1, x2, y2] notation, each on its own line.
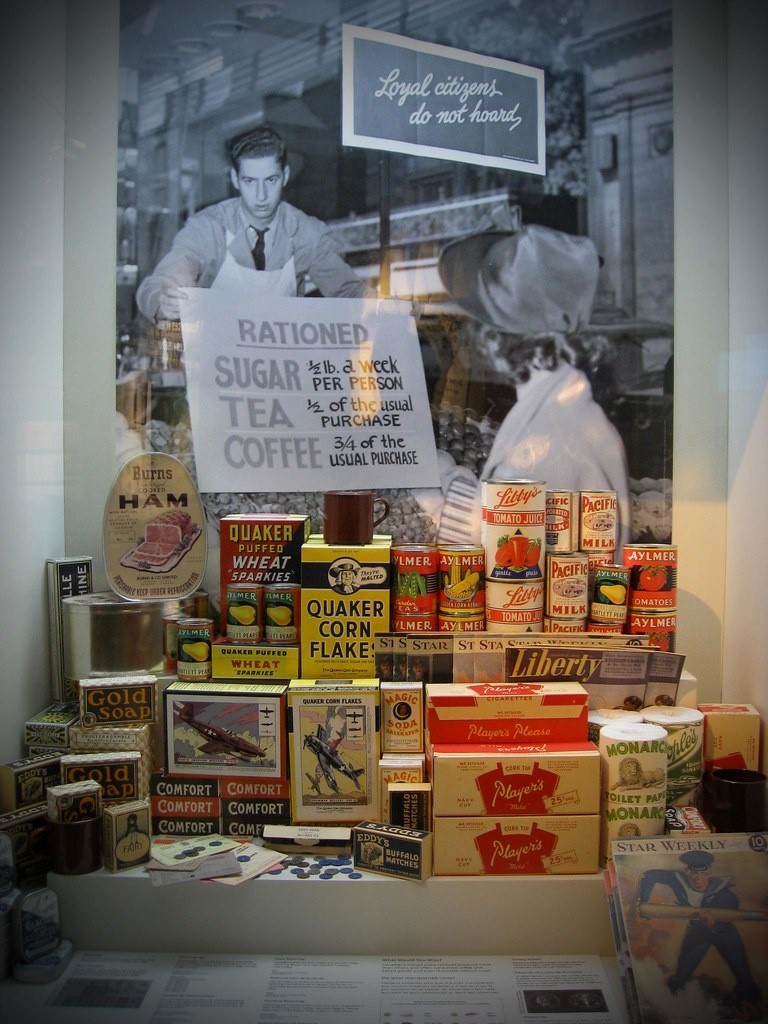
[44, 814, 104, 874]
[323, 491, 390, 545]
[703, 769, 768, 832]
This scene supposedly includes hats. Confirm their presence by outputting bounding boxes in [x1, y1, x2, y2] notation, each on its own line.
[438, 224, 599, 371]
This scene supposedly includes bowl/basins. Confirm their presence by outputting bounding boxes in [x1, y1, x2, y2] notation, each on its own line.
[62, 591, 209, 683]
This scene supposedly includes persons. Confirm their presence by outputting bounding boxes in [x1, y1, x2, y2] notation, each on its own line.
[135, 128, 381, 321]
[411, 222, 631, 548]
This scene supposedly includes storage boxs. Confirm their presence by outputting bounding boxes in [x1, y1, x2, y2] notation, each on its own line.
[0, 513, 760, 892]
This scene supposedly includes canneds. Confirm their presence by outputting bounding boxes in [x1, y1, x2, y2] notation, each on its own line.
[390, 476, 679, 652]
[163, 615, 215, 683]
[224, 582, 301, 644]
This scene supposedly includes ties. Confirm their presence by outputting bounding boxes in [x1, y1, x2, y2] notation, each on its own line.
[249, 225, 270, 271]
